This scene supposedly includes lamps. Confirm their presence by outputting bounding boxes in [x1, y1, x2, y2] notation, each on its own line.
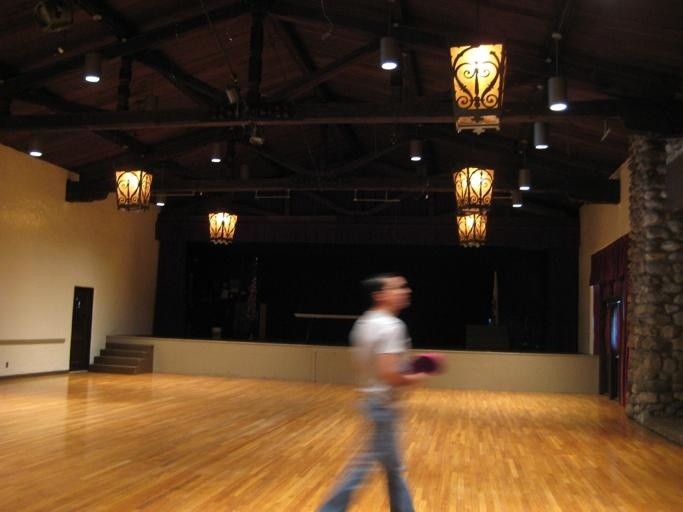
[409, 138, 426, 164]
[113, 130, 241, 248]
[377, 27, 402, 73]
[26, 132, 44, 158]
[445, 1, 573, 251]
[83, 47, 103, 85]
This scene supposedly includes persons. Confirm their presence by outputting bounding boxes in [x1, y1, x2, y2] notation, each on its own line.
[308, 272, 429, 512]
